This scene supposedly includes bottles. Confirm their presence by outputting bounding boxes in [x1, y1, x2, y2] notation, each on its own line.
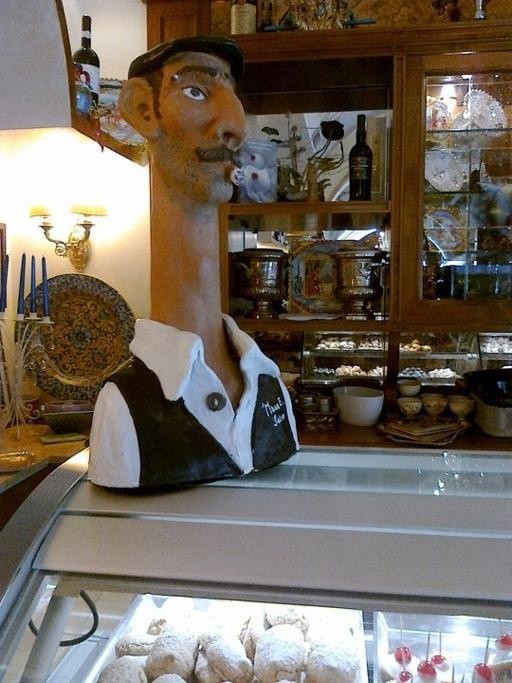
[72, 15, 100, 106]
[349, 112, 373, 201]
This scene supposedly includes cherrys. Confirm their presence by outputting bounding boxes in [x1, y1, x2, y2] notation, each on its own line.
[394, 615, 411, 666]
[399, 644, 414, 682]
[431, 631, 450, 672]
[495, 618, 511, 652]
[471, 636, 492, 682]
[418, 629, 437, 682]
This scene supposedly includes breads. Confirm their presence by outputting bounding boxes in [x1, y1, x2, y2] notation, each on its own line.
[95, 604, 362, 683]
[335, 364, 457, 379]
[316, 337, 432, 353]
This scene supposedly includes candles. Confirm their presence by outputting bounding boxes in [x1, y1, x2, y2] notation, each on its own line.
[2, 251, 53, 318]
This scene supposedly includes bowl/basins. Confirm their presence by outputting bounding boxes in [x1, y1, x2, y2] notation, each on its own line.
[397, 396, 423, 416]
[396, 379, 421, 396]
[42, 401, 94, 435]
[332, 386, 384, 427]
[421, 393, 448, 417]
[446, 396, 476, 417]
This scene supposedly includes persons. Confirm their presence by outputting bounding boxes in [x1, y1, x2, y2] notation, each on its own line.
[87, 34, 302, 489]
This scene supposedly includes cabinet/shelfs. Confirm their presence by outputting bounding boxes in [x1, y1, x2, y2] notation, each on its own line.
[198, 0, 512, 405]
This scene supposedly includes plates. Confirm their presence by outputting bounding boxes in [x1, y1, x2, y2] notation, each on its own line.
[425, 144, 467, 195]
[12, 273, 136, 405]
[423, 206, 467, 252]
[463, 89, 509, 138]
[287, 239, 353, 317]
[426, 96, 457, 145]
